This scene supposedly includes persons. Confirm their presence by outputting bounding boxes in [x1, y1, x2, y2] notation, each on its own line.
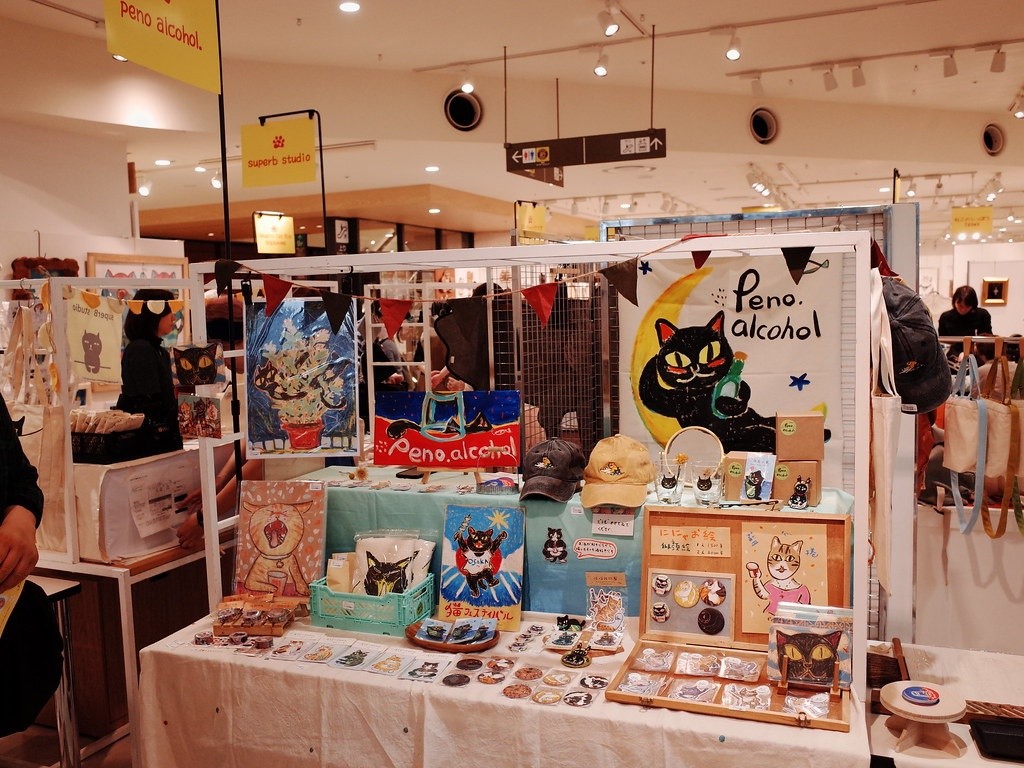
[965, 333, 1018, 398]
[117, 289, 178, 431]
[939, 286, 993, 374]
[357, 299, 402, 384]
[413, 327, 465, 391]
[0, 391, 44, 593]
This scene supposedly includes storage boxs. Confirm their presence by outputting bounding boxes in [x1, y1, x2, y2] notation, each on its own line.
[309, 573, 435, 637]
[776, 412, 825, 461]
[724, 451, 773, 502]
[71, 422, 183, 464]
[773, 461, 822, 506]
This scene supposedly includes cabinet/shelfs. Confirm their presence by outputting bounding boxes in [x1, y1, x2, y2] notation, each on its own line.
[0, 437, 870, 768]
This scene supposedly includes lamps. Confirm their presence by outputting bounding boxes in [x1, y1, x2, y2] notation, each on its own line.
[597, 0, 620, 37]
[544, 172, 1022, 246]
[593, 45, 609, 79]
[137, 174, 153, 198]
[723, 37, 1007, 99]
[211, 171, 223, 191]
[460, 69, 475, 94]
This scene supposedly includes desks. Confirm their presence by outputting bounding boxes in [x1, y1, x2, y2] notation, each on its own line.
[866, 639, 1024, 768]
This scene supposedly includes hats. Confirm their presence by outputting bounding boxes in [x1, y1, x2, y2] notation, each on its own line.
[581, 436, 655, 509]
[881, 281, 938, 417]
[519, 438, 586, 504]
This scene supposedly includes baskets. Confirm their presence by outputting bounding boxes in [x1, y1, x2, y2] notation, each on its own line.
[308, 572, 435, 636]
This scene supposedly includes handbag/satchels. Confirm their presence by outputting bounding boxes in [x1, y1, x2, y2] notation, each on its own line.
[935, 354, 1024, 538]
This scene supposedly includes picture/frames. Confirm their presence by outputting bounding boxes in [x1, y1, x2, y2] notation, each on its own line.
[980, 278, 1009, 306]
[87, 252, 191, 346]
